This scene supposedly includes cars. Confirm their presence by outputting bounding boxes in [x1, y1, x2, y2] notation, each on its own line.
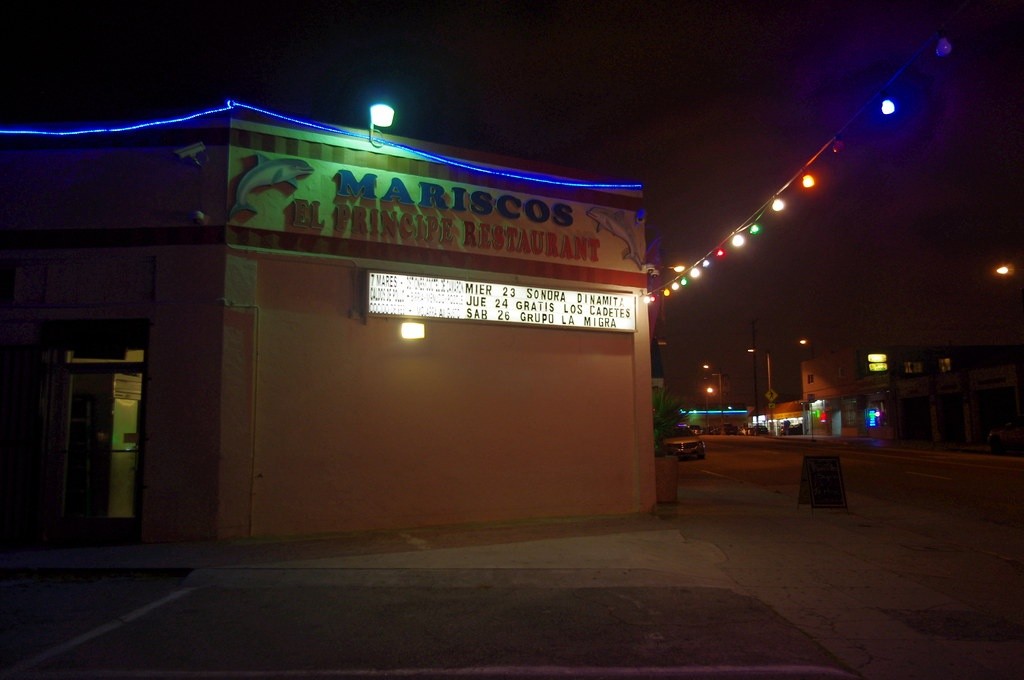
[750, 426, 769, 434]
[689, 423, 738, 435]
[658, 426, 705, 459]
[781, 424, 803, 436]
[986, 416, 1024, 456]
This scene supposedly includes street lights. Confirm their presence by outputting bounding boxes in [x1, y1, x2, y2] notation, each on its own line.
[705, 387, 714, 434]
[703, 365, 726, 435]
[748, 349, 774, 437]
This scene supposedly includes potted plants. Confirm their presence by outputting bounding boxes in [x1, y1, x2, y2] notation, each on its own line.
[652, 385, 691, 504]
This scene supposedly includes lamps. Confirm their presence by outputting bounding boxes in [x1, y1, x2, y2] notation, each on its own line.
[370, 103, 395, 148]
